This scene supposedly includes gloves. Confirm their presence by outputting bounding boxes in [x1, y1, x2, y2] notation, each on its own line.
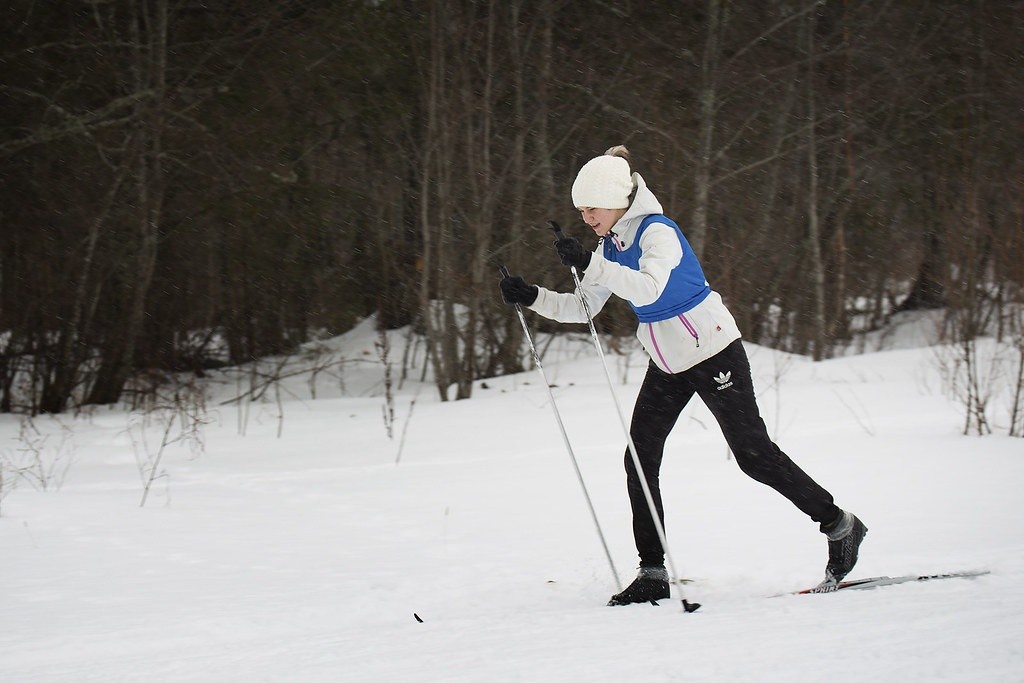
[499, 276, 538, 307]
[553, 237, 592, 271]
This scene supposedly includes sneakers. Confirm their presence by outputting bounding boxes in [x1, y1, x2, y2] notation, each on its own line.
[826, 514, 868, 584]
[611, 567, 670, 605]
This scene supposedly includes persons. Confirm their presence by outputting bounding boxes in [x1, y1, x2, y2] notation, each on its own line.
[500, 145, 868, 603]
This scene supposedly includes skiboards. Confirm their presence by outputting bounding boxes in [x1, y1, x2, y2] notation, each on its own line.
[412, 569, 994, 624]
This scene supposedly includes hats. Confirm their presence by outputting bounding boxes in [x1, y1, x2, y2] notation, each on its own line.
[571, 145, 634, 209]
